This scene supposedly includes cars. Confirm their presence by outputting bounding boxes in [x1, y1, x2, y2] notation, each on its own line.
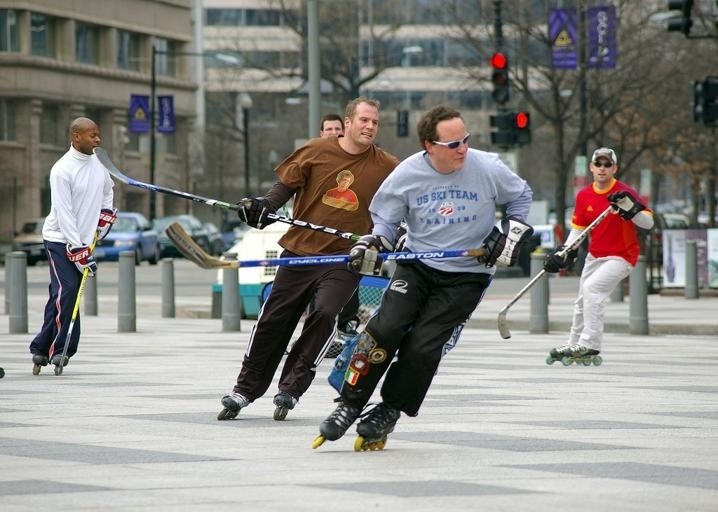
[528, 198, 717, 256]
[12, 213, 245, 265]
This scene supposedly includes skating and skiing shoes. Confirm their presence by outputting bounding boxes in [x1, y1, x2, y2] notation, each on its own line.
[353, 401, 401, 451]
[32, 354, 49, 375]
[217, 392, 250, 420]
[546, 345, 570, 365]
[52, 354, 69, 376]
[273, 391, 298, 420]
[562, 344, 602, 366]
[311, 398, 364, 450]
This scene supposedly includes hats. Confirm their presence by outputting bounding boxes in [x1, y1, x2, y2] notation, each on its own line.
[591, 146, 618, 164]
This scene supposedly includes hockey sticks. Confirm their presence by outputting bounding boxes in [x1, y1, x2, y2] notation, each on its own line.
[58, 231, 101, 374]
[497, 206, 614, 338]
[93, 147, 365, 243]
[164, 221, 494, 269]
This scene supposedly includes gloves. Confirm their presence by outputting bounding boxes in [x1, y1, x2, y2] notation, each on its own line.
[66, 242, 98, 278]
[606, 190, 646, 221]
[476, 214, 534, 269]
[394, 222, 409, 253]
[346, 235, 394, 276]
[543, 245, 579, 273]
[96, 207, 118, 240]
[236, 194, 277, 230]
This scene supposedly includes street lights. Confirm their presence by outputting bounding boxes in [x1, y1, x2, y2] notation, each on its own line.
[238, 94, 256, 199]
[353, 44, 424, 65]
[145, 42, 241, 224]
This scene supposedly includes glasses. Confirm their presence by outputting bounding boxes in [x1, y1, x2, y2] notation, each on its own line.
[592, 161, 613, 168]
[434, 132, 471, 149]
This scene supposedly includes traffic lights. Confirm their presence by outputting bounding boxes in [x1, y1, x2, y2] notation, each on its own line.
[489, 51, 506, 100]
[663, 0, 693, 33]
[488, 115, 509, 146]
[513, 112, 530, 144]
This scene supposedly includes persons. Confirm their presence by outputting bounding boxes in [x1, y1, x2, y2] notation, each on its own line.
[543, 146, 654, 360]
[222, 96, 402, 412]
[306, 112, 361, 355]
[319, 104, 534, 441]
[29, 116, 117, 367]
[321, 169, 359, 211]
